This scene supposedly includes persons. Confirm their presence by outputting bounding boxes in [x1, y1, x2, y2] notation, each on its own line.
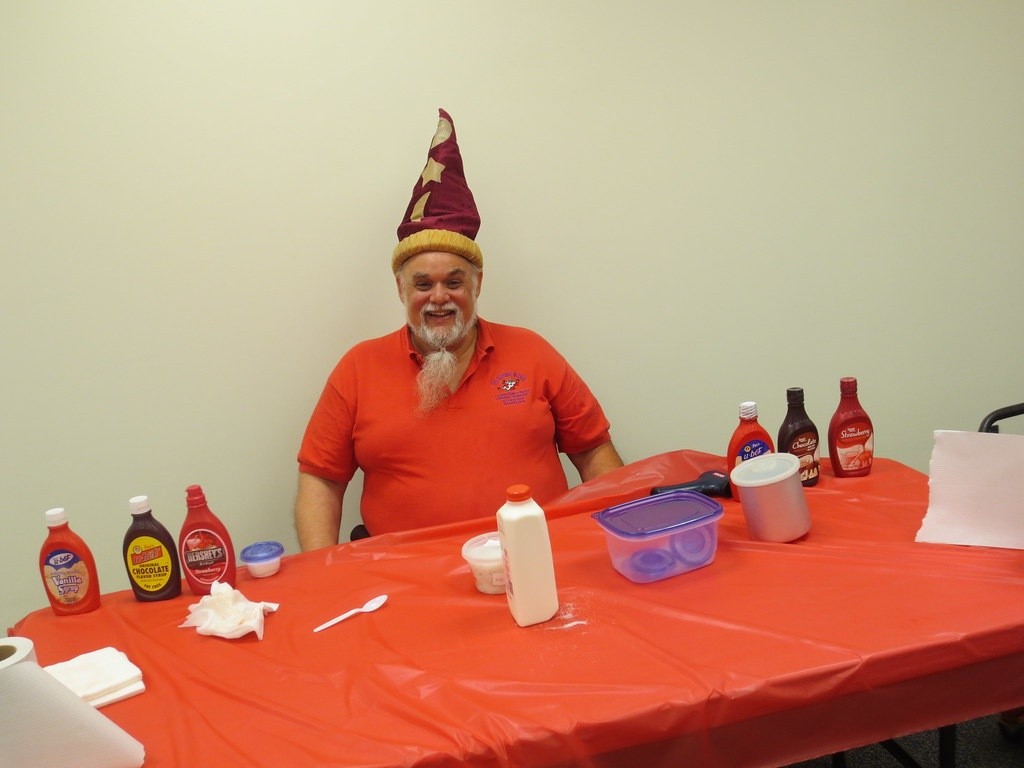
[294, 108, 625, 557]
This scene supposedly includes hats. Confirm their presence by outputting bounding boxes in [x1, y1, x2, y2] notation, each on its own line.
[392, 108, 484, 275]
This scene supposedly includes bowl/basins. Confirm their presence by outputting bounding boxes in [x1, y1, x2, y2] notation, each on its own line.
[593, 490, 724, 584]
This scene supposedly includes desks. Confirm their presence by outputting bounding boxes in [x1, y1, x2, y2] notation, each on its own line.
[7, 451, 1024, 768]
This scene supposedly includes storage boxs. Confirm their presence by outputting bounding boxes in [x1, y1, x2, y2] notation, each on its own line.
[590, 489, 724, 584]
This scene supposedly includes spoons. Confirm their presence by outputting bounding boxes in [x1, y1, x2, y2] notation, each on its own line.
[313, 594, 388, 634]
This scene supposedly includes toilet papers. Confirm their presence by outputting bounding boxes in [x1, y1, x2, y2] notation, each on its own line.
[0, 636, 38, 671]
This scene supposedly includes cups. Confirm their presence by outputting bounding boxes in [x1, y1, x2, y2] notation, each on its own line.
[239, 543, 284, 578]
[461, 535, 512, 594]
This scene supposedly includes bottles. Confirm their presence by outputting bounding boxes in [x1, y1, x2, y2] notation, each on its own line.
[495, 485, 560, 628]
[827, 377, 875, 477]
[726, 402, 776, 501]
[121, 495, 183, 601]
[181, 486, 235, 595]
[777, 387, 819, 487]
[730, 452, 813, 545]
[39, 507, 101, 615]
